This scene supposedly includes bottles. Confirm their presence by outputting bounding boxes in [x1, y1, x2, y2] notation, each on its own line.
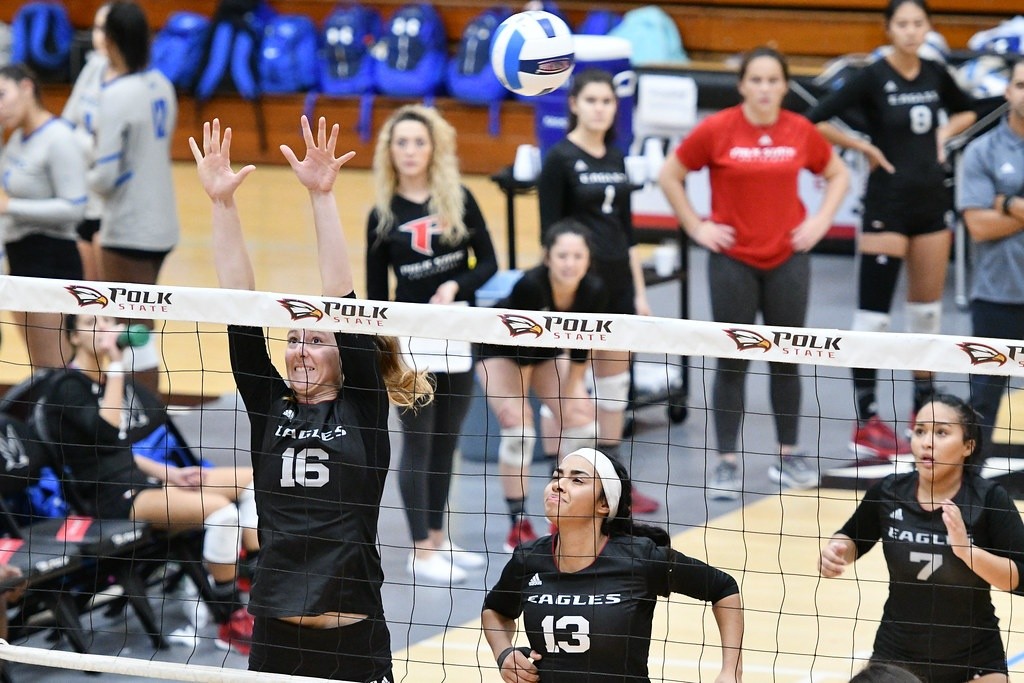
[117, 324, 149, 346]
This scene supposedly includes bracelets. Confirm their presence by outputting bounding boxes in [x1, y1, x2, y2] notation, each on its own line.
[107, 361, 123, 377]
[1002, 195, 1014, 215]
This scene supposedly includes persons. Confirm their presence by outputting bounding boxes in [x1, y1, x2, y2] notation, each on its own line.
[475, 222, 605, 551]
[809, 1, 983, 456]
[481, 448, 744, 683]
[60, 0, 179, 393]
[44, 313, 260, 654]
[537, 73, 659, 514]
[954, 53, 1023, 475]
[365, 104, 499, 582]
[187, 118, 434, 683]
[817, 393, 1024, 683]
[0, 63, 90, 369]
[659, 46, 853, 500]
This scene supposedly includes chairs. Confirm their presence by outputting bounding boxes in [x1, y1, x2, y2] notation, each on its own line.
[0, 367, 229, 683]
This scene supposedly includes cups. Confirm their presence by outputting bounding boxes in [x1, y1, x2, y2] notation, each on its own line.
[511, 144, 541, 181]
[624, 156, 648, 184]
[654, 248, 675, 276]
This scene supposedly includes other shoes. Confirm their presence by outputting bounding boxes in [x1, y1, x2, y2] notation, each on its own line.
[437, 540, 486, 568]
[848, 412, 912, 464]
[214, 607, 255, 657]
[628, 488, 659, 515]
[707, 461, 742, 499]
[234, 550, 261, 592]
[906, 410, 918, 435]
[407, 550, 467, 583]
[503, 513, 536, 555]
[768, 452, 821, 490]
